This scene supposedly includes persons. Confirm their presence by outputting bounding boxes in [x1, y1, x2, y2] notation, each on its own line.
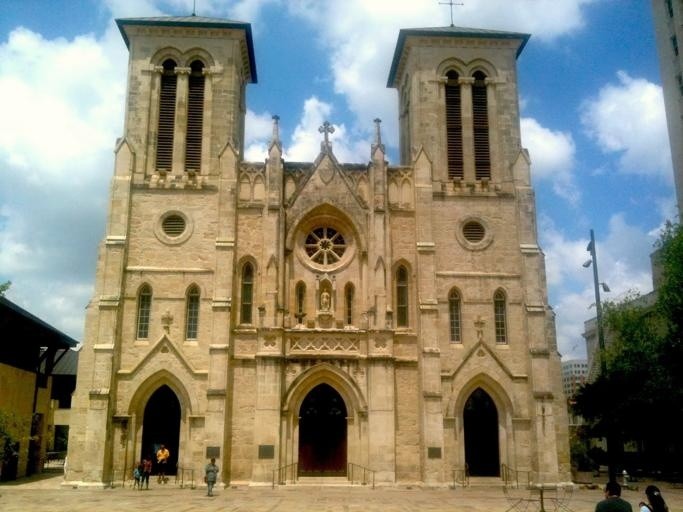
[203, 457, 220, 497]
[132, 441, 170, 491]
[639, 485, 669, 511]
[596, 481, 632, 511]
[320, 288, 329, 309]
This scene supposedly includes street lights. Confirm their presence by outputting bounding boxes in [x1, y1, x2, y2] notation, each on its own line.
[581, 227, 614, 493]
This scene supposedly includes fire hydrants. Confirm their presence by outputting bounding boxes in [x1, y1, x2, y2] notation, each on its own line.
[621, 469, 629, 486]
